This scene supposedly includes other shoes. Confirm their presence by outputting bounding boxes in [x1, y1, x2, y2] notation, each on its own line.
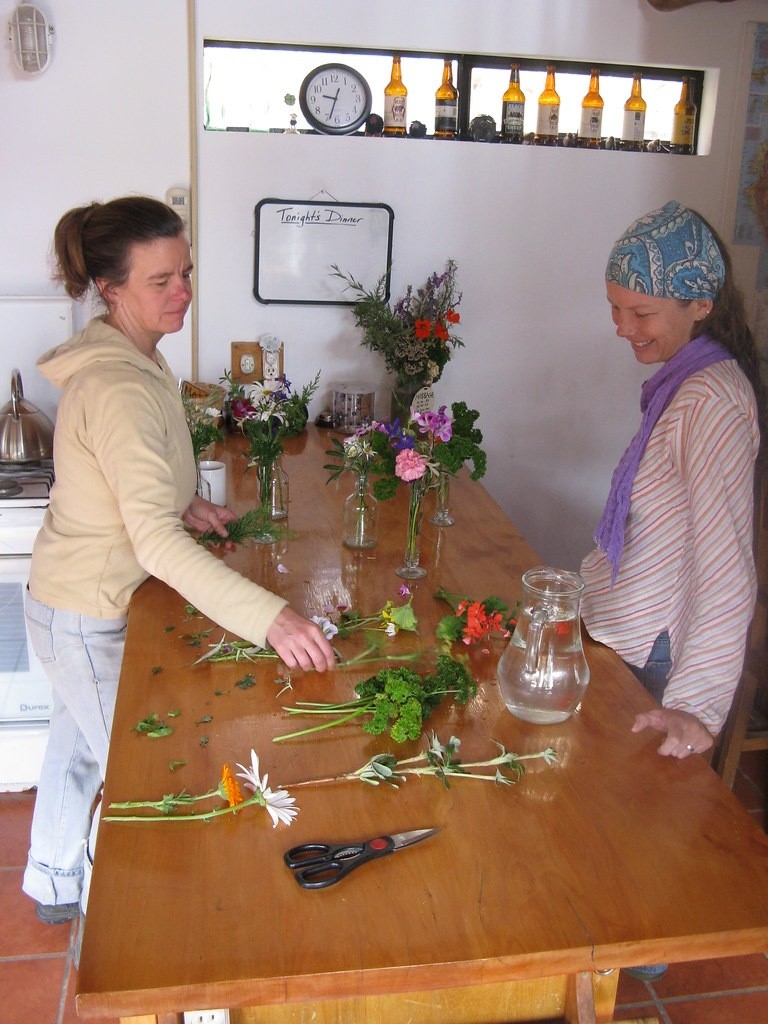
[36, 903, 79, 924]
[627, 971, 669, 982]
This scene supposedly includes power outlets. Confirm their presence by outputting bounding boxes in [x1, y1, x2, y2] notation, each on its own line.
[262, 350, 279, 379]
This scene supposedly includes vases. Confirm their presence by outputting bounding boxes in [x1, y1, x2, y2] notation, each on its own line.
[342, 477, 379, 548]
[428, 466, 455, 526]
[395, 489, 427, 579]
[391, 374, 432, 437]
[256, 453, 289, 518]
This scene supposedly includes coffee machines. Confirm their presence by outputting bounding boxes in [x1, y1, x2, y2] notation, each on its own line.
[502, 63, 526, 144]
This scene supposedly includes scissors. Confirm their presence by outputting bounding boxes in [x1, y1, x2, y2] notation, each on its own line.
[284, 827, 443, 890]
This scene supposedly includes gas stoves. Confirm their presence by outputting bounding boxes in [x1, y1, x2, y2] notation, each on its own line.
[0, 295, 74, 507]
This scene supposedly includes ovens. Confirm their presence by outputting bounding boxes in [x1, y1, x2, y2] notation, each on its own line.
[0, 507, 53, 792]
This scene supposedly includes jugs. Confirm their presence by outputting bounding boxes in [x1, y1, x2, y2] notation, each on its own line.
[497, 566, 590, 724]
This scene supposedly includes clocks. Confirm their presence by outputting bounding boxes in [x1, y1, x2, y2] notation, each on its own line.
[299, 63, 371, 136]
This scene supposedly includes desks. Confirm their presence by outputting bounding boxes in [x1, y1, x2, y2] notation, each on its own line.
[74, 424, 768, 1024]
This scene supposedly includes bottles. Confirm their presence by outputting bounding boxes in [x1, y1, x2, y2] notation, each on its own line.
[577, 68, 604, 149]
[534, 66, 560, 146]
[620, 72, 646, 153]
[670, 76, 697, 155]
[435, 58, 457, 137]
[384, 56, 408, 137]
[283, 114, 300, 135]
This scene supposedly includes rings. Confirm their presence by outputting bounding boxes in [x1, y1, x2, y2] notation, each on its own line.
[686, 744, 694, 752]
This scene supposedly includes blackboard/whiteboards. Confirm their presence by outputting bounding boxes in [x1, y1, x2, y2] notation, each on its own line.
[254, 199, 394, 304]
[0, 0, 197, 303]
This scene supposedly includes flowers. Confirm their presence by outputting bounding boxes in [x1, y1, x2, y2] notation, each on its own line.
[324, 402, 488, 502]
[102, 749, 301, 828]
[219, 369, 321, 473]
[329, 260, 466, 387]
[432, 586, 518, 646]
[207, 585, 417, 659]
[108, 764, 241, 816]
[180, 387, 224, 468]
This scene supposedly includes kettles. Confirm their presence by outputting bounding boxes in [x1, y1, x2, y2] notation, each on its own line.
[0, 369, 55, 462]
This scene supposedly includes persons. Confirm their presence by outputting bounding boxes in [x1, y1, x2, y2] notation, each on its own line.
[575, 200, 761, 760]
[20, 198, 334, 971]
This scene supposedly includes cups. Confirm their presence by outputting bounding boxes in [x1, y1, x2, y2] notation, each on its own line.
[329, 380, 375, 434]
[198, 461, 226, 508]
